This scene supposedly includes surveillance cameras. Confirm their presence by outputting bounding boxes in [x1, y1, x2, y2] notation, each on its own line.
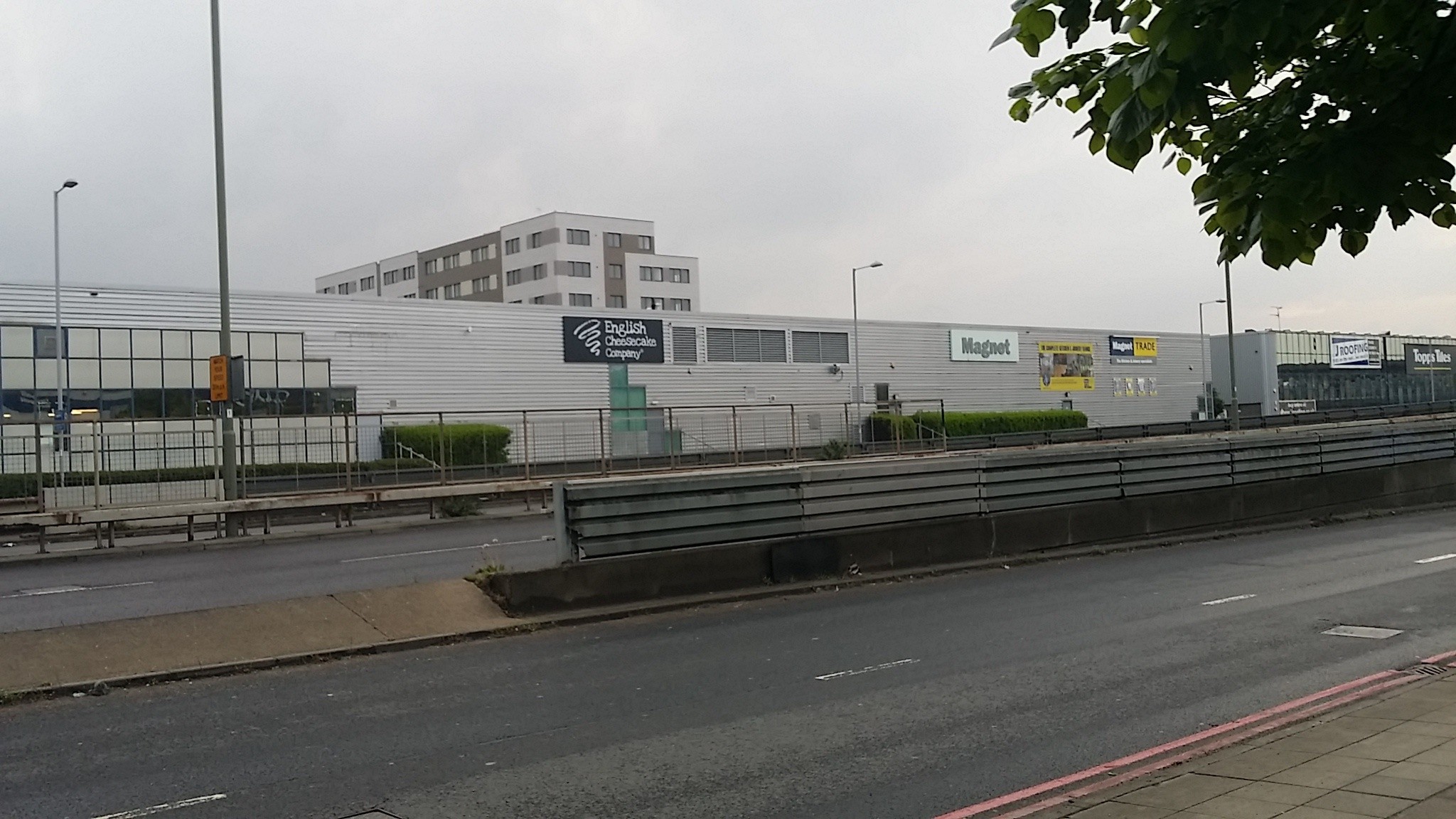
[830, 363, 840, 374]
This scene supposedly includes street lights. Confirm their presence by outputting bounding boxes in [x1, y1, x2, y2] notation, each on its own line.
[853, 262, 883, 443]
[55, 177, 79, 489]
[1200, 300, 1226, 420]
